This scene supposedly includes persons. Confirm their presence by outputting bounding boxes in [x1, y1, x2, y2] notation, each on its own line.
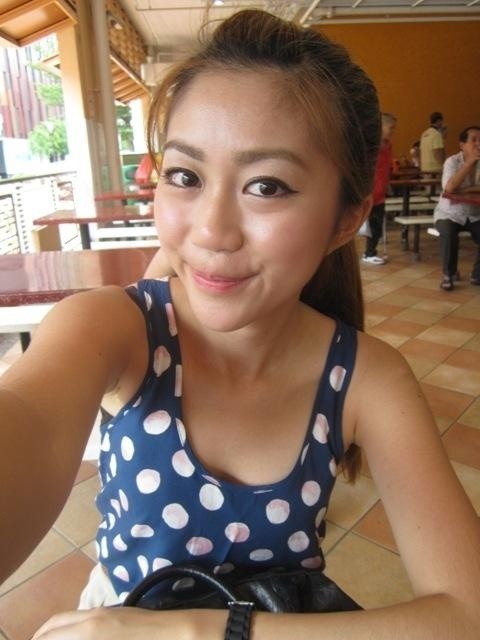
[2, 4, 479, 640]
[404, 141, 419, 168]
[419, 110, 448, 229]
[362, 112, 399, 265]
[432, 124, 480, 290]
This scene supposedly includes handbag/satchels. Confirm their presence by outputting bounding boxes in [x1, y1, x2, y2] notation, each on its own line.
[107, 560, 362, 611]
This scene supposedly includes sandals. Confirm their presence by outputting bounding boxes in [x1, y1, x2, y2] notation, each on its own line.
[441, 276, 453, 290]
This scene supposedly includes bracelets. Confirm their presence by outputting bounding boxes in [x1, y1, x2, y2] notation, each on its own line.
[224, 600, 253, 640]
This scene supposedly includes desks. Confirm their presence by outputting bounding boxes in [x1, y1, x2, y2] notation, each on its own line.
[0, 177, 176, 358]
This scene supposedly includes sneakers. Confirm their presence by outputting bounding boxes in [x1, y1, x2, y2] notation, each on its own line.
[361, 253, 385, 264]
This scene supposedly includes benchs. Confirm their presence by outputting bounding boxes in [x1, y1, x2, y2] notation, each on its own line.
[377, 157, 480, 281]
